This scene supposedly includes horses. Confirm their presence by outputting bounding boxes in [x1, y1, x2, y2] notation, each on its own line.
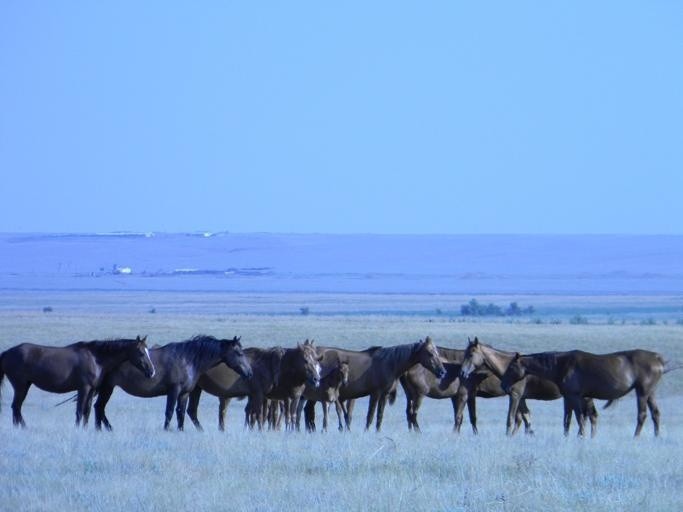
[1, 332, 683, 440]
